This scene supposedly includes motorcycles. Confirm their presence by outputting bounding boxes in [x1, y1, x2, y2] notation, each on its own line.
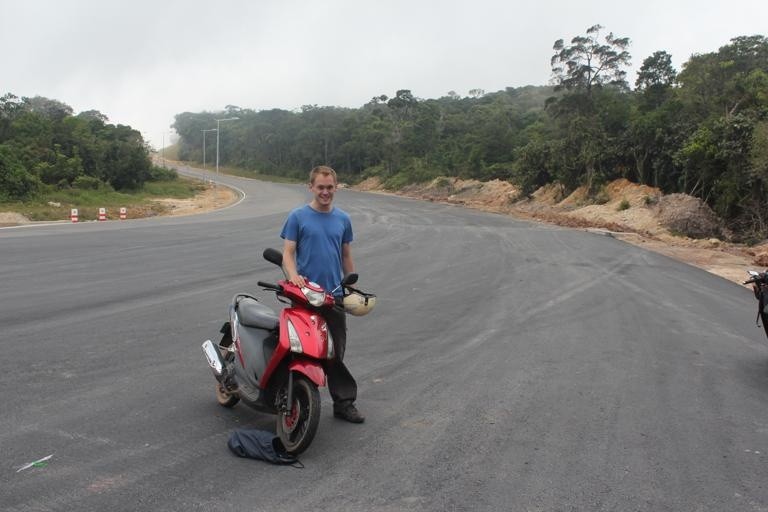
[740, 268, 768, 339]
[200, 246, 359, 459]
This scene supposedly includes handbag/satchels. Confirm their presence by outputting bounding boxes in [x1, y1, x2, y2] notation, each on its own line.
[227, 428, 297, 466]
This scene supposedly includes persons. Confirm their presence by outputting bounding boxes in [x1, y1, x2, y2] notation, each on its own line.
[281, 165, 365, 423]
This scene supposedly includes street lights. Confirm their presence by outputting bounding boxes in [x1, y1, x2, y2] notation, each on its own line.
[200, 128, 217, 169]
[215, 116, 240, 176]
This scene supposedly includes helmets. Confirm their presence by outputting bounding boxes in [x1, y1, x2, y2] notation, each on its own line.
[343, 289, 377, 316]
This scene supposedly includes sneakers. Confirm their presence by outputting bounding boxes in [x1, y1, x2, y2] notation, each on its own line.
[334, 404, 365, 424]
